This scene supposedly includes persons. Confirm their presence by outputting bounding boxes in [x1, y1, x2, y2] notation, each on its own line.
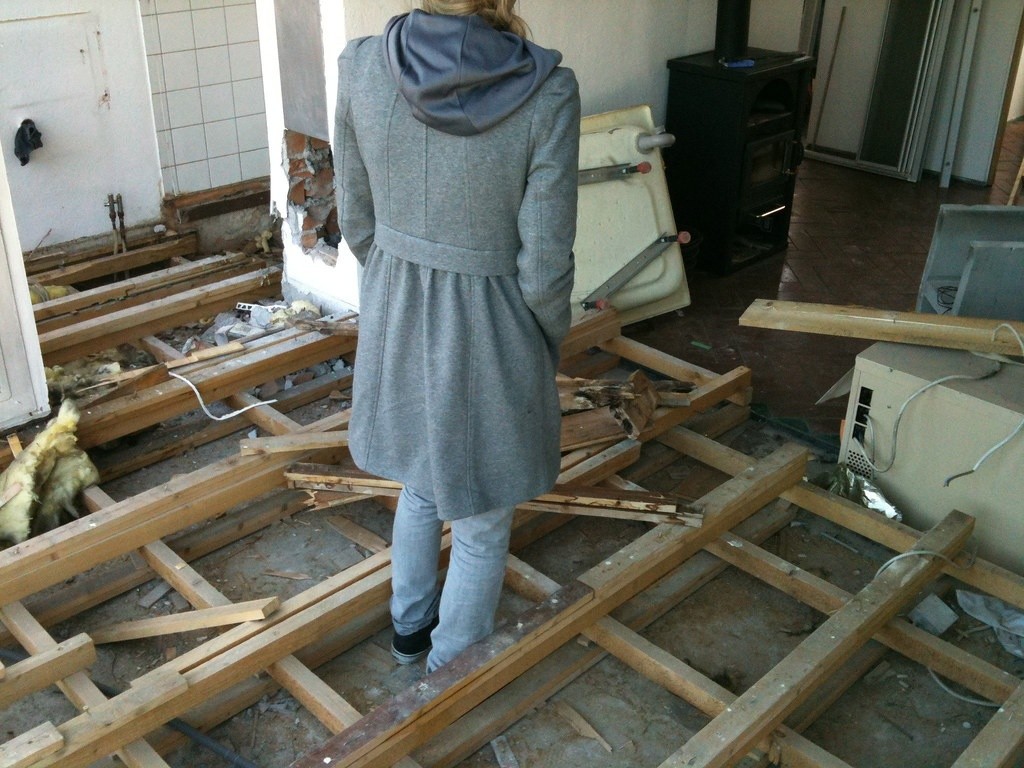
[333, 1, 581, 676]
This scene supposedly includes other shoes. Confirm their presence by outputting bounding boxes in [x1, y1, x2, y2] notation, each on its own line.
[390, 617, 441, 664]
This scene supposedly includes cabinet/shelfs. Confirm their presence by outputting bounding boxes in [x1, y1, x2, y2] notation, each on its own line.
[662, 47, 815, 281]
